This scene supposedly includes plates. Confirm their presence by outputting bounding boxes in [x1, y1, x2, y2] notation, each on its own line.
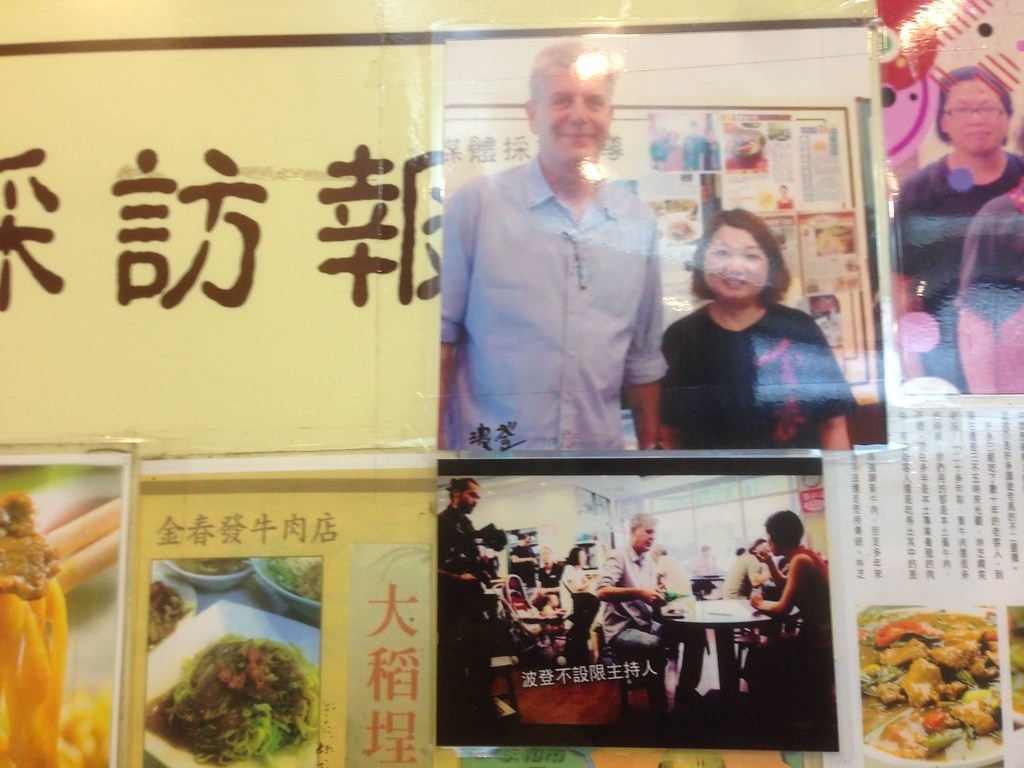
[144, 598, 318, 768]
[149, 560, 198, 651]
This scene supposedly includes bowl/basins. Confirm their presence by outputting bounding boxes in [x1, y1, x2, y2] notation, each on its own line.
[860, 604, 1004, 768]
[161, 558, 255, 588]
[249, 556, 322, 622]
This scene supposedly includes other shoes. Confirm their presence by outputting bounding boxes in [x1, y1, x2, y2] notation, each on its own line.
[674, 685, 704, 711]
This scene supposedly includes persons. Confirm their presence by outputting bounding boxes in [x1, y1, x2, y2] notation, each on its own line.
[438, 476, 832, 731]
[436, 42, 669, 450]
[777, 186, 793, 209]
[889, 65, 1024, 393]
[659, 208, 859, 452]
[660, 121, 720, 170]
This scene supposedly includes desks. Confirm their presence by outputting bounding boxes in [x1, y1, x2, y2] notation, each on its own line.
[656, 596, 774, 694]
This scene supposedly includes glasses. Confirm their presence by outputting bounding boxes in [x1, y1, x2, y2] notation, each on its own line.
[563, 228, 593, 289]
[943, 106, 1007, 121]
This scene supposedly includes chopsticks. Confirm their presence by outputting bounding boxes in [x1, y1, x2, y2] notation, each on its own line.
[40, 497, 123, 591]
